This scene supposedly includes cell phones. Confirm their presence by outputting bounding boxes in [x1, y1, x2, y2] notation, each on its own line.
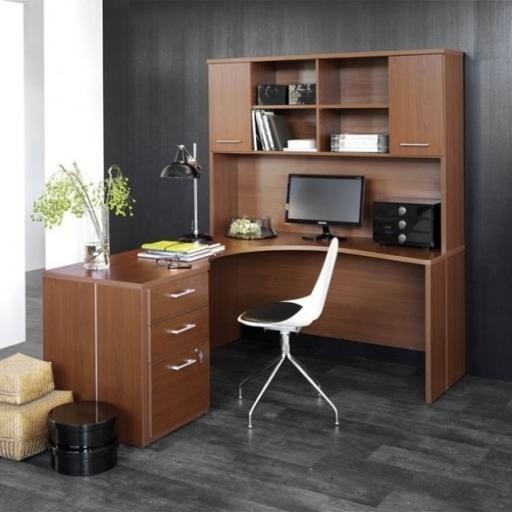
[168, 263, 191, 269]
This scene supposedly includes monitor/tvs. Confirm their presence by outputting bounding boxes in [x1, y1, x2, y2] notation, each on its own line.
[284, 175, 365, 242]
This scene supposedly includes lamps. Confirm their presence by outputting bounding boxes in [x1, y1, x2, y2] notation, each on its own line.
[161, 143, 213, 244]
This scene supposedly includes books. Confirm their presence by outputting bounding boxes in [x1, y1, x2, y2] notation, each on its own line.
[137, 240, 226, 262]
[251, 109, 318, 152]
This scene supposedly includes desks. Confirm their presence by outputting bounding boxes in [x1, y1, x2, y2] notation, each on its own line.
[44, 228, 467, 449]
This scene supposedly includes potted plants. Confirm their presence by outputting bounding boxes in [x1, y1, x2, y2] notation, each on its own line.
[30, 162, 135, 270]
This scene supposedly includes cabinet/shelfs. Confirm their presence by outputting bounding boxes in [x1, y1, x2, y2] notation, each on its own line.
[205, 49, 464, 253]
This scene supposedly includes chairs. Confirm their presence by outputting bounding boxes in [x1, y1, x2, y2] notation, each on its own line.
[238, 238, 339, 428]
